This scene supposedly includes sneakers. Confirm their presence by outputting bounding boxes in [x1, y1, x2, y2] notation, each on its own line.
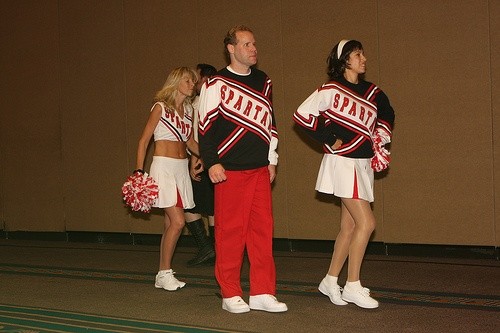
[248, 294, 288, 312]
[155, 269, 186, 291]
[318, 278, 348, 305]
[342, 285, 379, 307]
[222, 296, 250, 313]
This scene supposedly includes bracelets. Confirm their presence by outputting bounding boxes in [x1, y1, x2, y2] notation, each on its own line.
[133, 169, 144, 175]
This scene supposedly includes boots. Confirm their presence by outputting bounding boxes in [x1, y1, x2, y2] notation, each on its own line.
[185, 218, 216, 266]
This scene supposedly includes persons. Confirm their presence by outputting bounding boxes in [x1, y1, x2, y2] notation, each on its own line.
[292, 39, 395, 308]
[132, 63, 217, 291]
[198, 25, 288, 314]
